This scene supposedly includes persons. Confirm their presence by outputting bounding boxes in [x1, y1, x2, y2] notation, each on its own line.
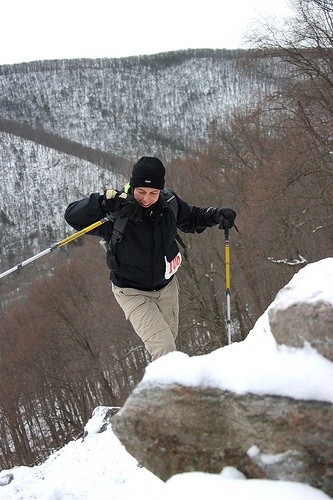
[64, 157, 236, 362]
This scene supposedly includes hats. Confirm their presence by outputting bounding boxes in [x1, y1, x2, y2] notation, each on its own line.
[130, 157, 166, 190]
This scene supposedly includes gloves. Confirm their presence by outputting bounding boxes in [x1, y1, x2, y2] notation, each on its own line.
[203, 207, 236, 230]
[104, 189, 140, 219]
